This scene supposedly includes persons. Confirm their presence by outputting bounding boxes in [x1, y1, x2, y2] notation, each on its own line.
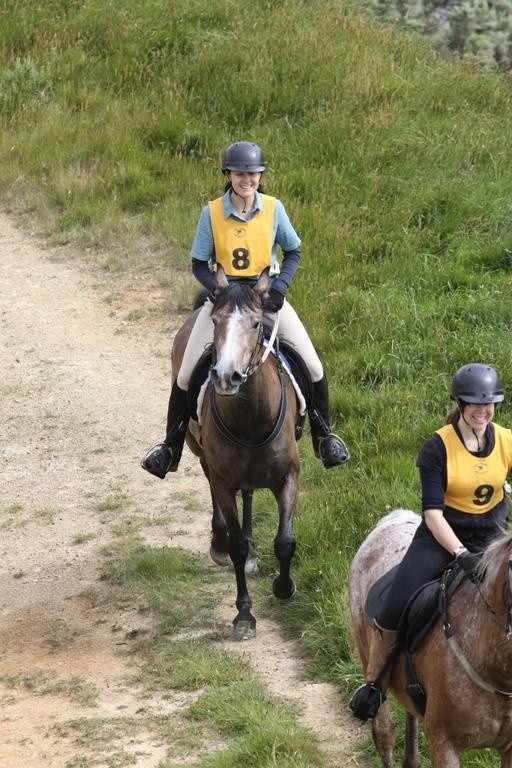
[137, 139, 352, 480]
[345, 360, 511, 722]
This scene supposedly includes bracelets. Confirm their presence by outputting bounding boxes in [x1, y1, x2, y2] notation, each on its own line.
[453, 546, 466, 555]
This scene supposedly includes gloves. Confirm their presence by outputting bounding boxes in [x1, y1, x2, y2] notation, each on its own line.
[264, 288, 284, 311]
[457, 551, 487, 584]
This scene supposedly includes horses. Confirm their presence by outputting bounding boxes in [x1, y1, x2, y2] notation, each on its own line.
[169, 260, 303, 643]
[345, 479, 512, 768]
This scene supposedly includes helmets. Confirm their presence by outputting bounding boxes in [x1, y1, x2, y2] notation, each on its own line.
[450, 362, 505, 404]
[221, 142, 266, 173]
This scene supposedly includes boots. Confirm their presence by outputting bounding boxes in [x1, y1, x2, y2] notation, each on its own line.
[144, 445, 179, 475]
[311, 436, 348, 462]
[355, 686, 387, 719]
